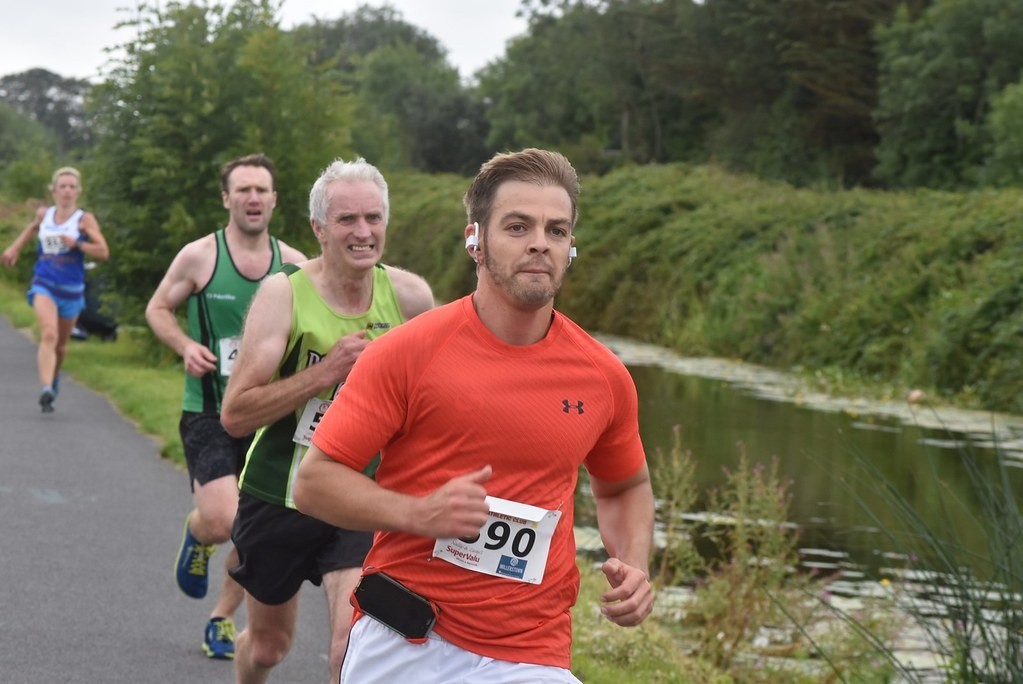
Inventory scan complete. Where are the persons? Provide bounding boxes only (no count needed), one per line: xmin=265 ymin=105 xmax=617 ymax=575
xmin=1 ymin=167 xmax=110 ymax=413
xmin=143 ymin=152 xmax=309 ymax=660
xmin=290 ymin=146 xmax=654 ymax=684
xmin=220 ymin=159 xmax=432 ymax=684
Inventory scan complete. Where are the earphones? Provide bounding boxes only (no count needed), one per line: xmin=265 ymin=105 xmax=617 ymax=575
xmin=468 ymin=223 xmax=479 ymax=253
xmin=569 ymin=244 xmax=577 ymax=264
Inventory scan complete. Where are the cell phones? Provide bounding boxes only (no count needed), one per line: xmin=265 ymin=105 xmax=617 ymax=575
xmin=349 ymin=567 xmax=440 ymax=645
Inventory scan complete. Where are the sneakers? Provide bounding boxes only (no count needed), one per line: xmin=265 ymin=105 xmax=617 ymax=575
xmin=173 ymin=510 xmax=217 ymax=601
xmin=37 ymin=387 xmax=55 ymax=412
xmin=51 ymin=376 xmax=62 ymax=398
xmin=202 ymin=619 xmax=237 ymax=660
